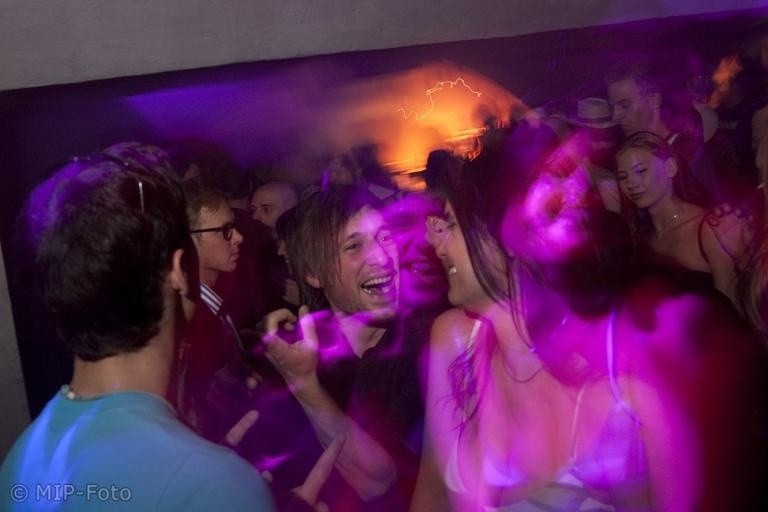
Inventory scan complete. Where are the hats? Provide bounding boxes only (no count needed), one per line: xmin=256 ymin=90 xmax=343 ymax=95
xmin=567 ymin=97 xmax=618 ymax=130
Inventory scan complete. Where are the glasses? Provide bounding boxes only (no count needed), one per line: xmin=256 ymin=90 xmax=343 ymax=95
xmin=73 ymin=150 xmax=156 ymax=212
xmin=191 ymin=223 xmax=233 ymax=241
